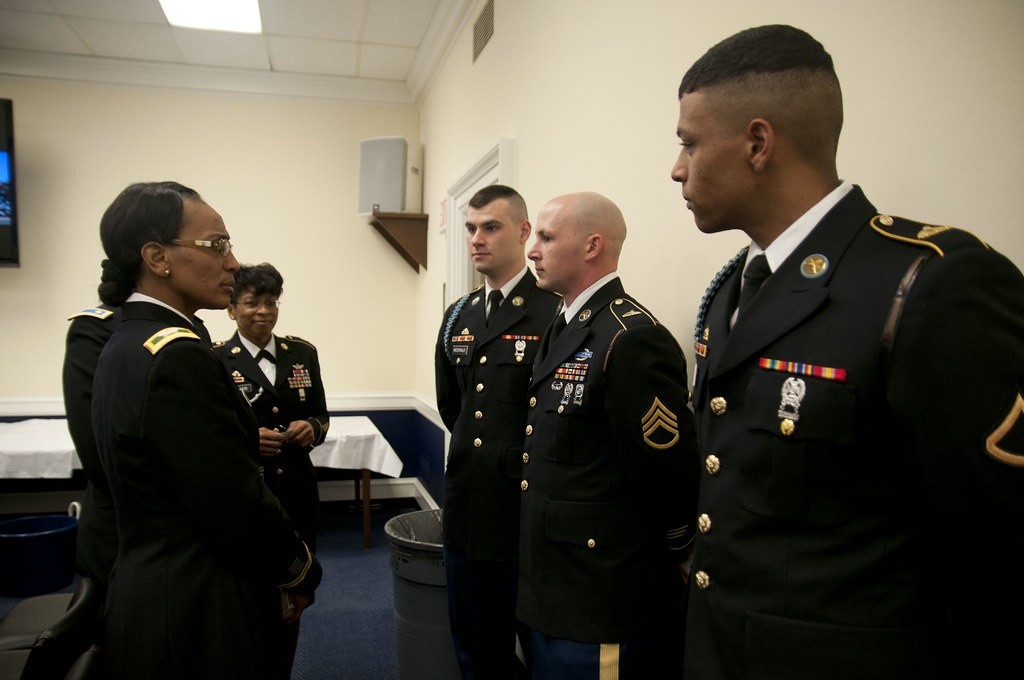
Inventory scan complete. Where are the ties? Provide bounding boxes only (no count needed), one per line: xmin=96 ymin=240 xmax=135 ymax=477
xmin=547 ymin=311 xmax=567 ymax=356
xmin=486 ymin=289 xmax=504 ymax=328
xmin=739 ymin=254 xmax=770 ymax=320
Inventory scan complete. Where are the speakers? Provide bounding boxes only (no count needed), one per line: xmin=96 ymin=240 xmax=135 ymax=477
xmin=357 ymin=136 xmax=424 ymax=216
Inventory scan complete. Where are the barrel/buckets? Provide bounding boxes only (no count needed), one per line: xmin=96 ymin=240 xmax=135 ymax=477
xmin=0 ymin=502 xmax=82 ymax=595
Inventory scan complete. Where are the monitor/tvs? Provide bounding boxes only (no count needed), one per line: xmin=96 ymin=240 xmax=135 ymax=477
xmin=0 ymin=98 xmax=21 ymax=267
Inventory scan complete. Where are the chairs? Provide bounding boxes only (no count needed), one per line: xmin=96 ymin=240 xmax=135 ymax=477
xmin=1 ymin=573 xmax=105 ymax=680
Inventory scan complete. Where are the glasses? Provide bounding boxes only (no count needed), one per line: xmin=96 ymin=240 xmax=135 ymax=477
xmin=235 ymin=299 xmax=282 ymax=311
xmin=163 ymin=236 xmax=231 ymax=256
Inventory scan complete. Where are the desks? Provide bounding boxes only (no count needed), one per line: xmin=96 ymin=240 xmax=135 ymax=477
xmin=1 ymin=411 xmax=402 ymax=551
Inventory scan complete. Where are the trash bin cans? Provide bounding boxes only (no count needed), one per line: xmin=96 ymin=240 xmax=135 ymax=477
xmin=0 ymin=515 xmax=82 ymax=624
xmin=384 ymin=507 xmax=526 ymax=680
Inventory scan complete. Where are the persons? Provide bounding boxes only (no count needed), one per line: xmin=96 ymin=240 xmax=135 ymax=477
xmin=92 ymin=181 xmax=324 ymax=679
xmin=435 ymin=184 xmax=563 ymax=680
xmin=672 ymin=25 xmax=1024 ymax=679
xmin=515 ymin=190 xmax=690 ymax=680
xmin=212 ymin=263 xmax=330 ymax=556
xmin=62 ymin=306 xmax=121 ymax=596
